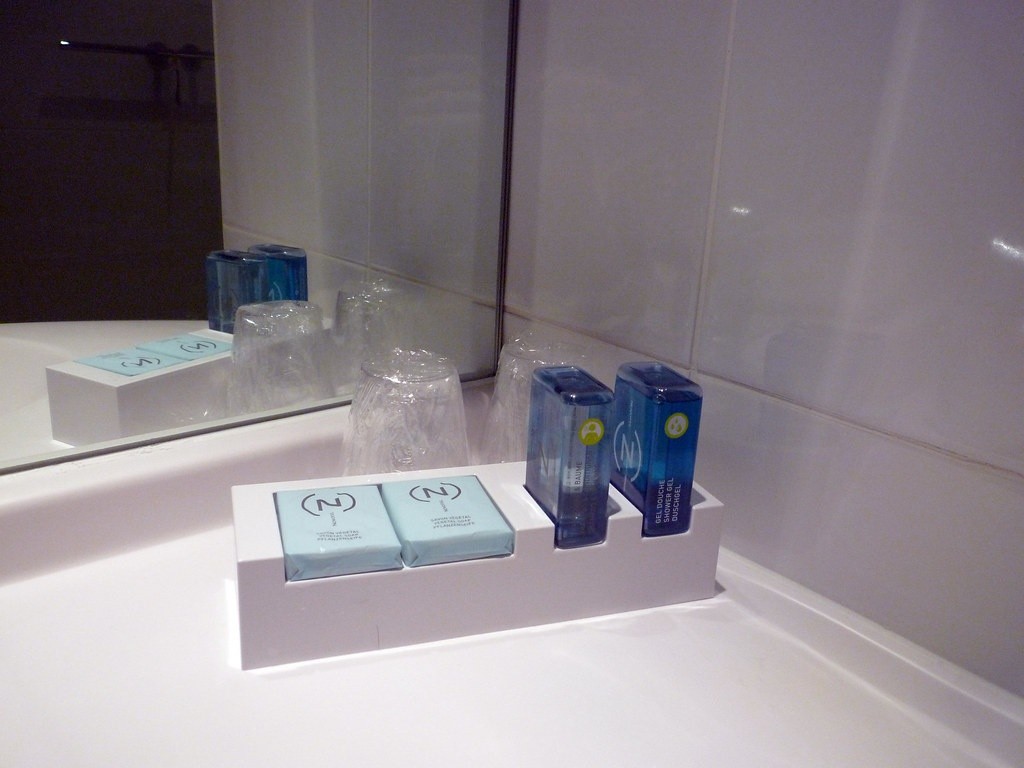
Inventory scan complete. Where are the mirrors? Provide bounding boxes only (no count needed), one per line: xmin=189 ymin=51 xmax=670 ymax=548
xmin=0 ymin=0 xmax=523 ymax=476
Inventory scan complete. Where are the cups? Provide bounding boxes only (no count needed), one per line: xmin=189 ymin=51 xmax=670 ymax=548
xmin=230 ymin=301 xmax=339 ymax=415
xmin=335 ymin=280 xmax=424 ymax=391
xmin=479 ymin=339 xmax=594 ymax=464
xmin=342 ymin=351 xmax=473 ymax=475
